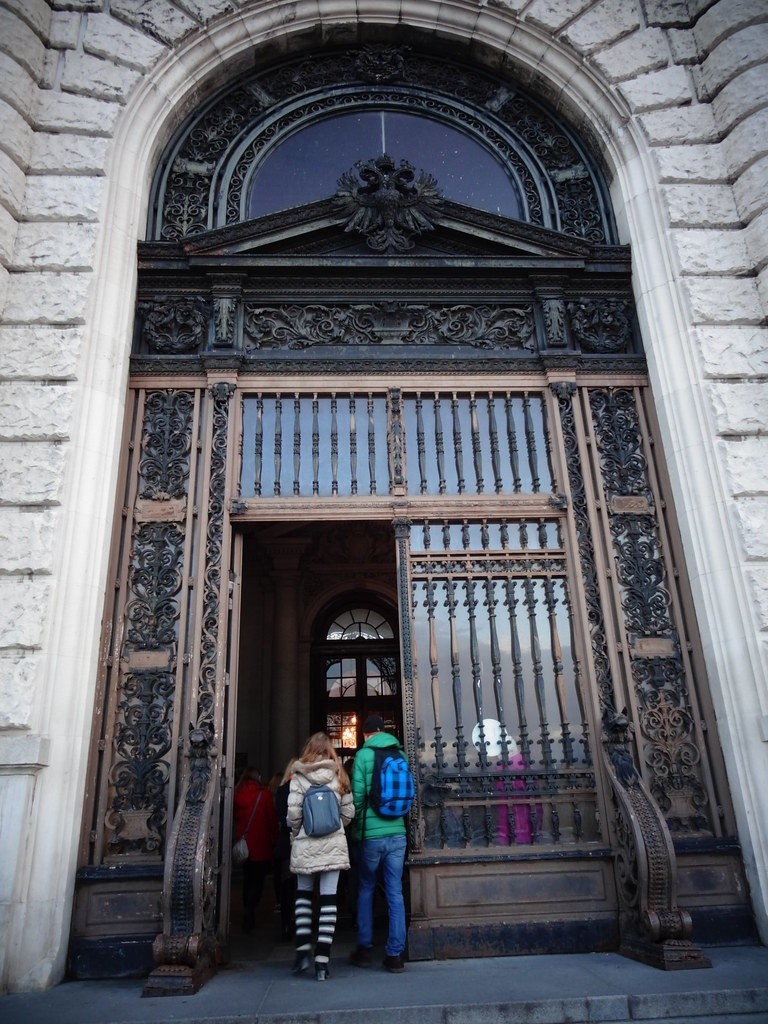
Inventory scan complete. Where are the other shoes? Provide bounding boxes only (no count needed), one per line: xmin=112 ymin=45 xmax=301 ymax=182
xmin=383 ymin=956 xmax=405 ymax=973
xmin=351 ymin=950 xmax=371 ymax=967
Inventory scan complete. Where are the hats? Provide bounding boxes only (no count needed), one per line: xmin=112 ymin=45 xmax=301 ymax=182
xmin=362 ymin=716 xmax=384 ymax=733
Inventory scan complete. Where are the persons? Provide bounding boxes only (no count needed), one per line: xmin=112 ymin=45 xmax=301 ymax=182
xmin=234 ymin=761 xmax=278 ymax=932
xmin=350 ymin=716 xmax=408 ymax=973
xmin=280 ymin=733 xmax=356 ymax=982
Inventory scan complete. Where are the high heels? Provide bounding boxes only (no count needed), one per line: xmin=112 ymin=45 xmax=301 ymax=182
xmin=298 ymin=951 xmax=311 ymax=970
xmin=314 ymin=962 xmax=330 ymax=981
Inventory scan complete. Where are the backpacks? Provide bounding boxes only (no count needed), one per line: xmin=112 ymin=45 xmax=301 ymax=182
xmin=302 ymin=784 xmax=341 ymax=838
xmin=366 ymin=744 xmax=415 ymax=820
